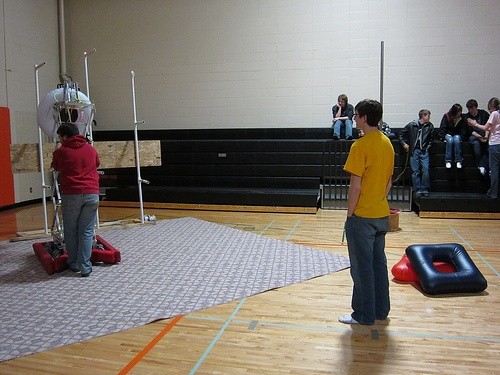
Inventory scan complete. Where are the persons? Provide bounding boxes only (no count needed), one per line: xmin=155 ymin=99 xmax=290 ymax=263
xmin=332 ymin=94 xmax=355 ymax=140
xmin=399 ymin=109 xmax=435 ymax=197
xmin=438 ymin=103 xmax=466 ymax=169
xmin=463 ymin=98 xmax=491 ymax=175
xmin=337 ymin=99 xmax=396 ymax=325
xmin=49 ymin=123 xmax=100 ymax=278
xmin=467 ymin=96 xmax=500 ymax=199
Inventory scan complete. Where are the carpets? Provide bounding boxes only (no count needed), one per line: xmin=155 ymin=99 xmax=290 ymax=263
xmin=0 ymin=217 xmax=350 ymax=363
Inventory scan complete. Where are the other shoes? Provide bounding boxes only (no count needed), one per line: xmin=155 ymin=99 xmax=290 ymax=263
xmin=333 ymin=135 xmax=340 ymax=140
xmin=81 ymin=267 xmax=92 ymax=277
xmin=346 ymin=135 xmax=353 ymax=140
xmin=478 ymin=167 xmax=487 ymax=176
xmin=338 ymin=314 xmax=359 ymax=324
xmin=446 ymin=161 xmax=451 ymax=168
xmin=457 ymin=162 xmax=462 ymax=168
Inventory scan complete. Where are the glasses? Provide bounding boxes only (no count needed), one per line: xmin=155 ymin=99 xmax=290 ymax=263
xmin=352 ymin=113 xmax=359 ymax=118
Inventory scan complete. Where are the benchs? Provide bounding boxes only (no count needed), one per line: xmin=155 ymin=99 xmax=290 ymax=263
xmin=90 ymin=127 xmax=500 ymax=212
